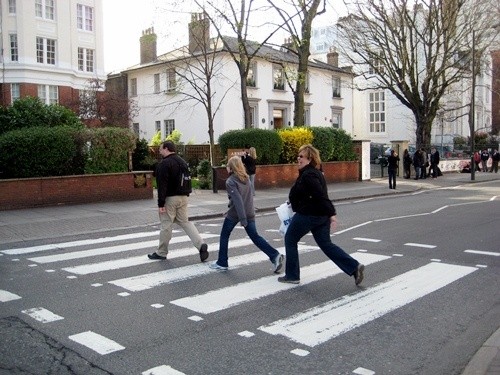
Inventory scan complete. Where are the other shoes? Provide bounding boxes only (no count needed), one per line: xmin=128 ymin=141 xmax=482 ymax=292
xmin=273 ymin=253 xmax=284 ymax=272
xmin=148 ymin=251 xmax=167 ymax=259
xmin=200 ymin=242 xmax=209 ymax=261
xmin=278 ymin=274 xmax=300 ymax=284
xmin=208 ymin=260 xmax=228 ymax=271
xmin=354 ymin=263 xmax=364 ymax=285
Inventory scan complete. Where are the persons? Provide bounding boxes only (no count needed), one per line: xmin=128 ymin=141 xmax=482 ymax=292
xmin=241 ymin=146 xmax=257 ymax=196
xmin=277 ymin=144 xmax=366 ymax=285
xmin=207 ymin=156 xmax=285 ymax=274
xmin=148 ymin=140 xmax=209 ymax=263
xmin=463 ymin=148 xmax=500 ymax=173
xmin=402 ymin=148 xmax=413 ymax=179
xmin=387 ymin=149 xmax=400 ymax=190
xmin=412 ymin=147 xmax=443 ymax=181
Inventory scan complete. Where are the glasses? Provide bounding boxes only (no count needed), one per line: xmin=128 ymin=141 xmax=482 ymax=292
xmin=296 ymin=154 xmax=306 ymax=158
